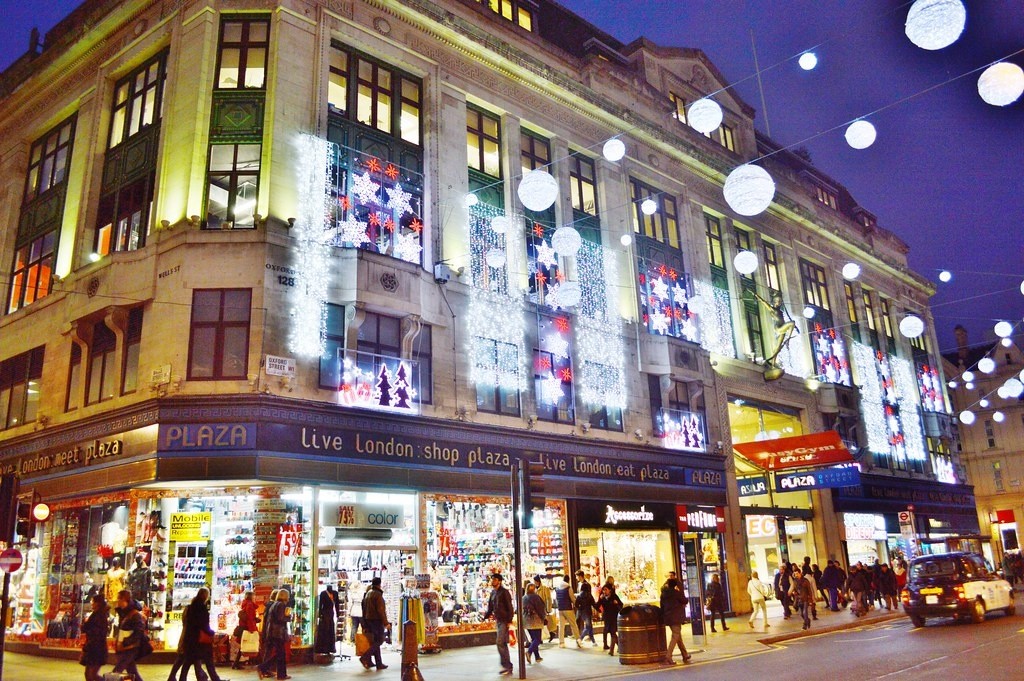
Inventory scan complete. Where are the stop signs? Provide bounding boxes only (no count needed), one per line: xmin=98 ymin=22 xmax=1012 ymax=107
xmin=0 ymin=548 xmax=22 ymax=574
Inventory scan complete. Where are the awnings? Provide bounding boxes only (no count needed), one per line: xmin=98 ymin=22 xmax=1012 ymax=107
xmin=732 ymin=430 xmax=853 ymax=472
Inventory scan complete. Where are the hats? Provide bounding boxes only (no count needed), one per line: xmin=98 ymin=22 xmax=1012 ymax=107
xmin=534 ymin=575 xmax=541 ymax=584
xmin=581 ymin=583 xmax=590 ymax=592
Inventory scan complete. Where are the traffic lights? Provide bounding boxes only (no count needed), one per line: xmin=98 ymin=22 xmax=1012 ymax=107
xmin=16 ymin=503 xmax=36 ymax=548
xmin=33 ymin=503 xmax=50 ymax=521
xmin=519 ymin=456 xmax=548 ymax=531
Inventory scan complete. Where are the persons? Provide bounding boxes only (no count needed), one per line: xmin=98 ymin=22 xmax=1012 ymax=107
xmin=106 ymin=553 xmax=150 ymax=605
xmin=79 ymin=595 xmax=108 ymax=681
xmin=774 ymin=556 xmax=907 ymax=631
xmin=746 ymin=571 xmax=770 ymax=628
xmin=232 ymin=589 xmax=293 ymax=680
xmin=1001 ymin=550 xmax=1024 ymax=587
xmin=166 ymin=588 xmax=231 ymax=681
xmin=315 ymin=585 xmax=340 ymax=653
xmin=522 ymin=570 xmax=624 ymax=663
xmin=706 ymin=574 xmax=729 ymax=632
xmin=111 ymin=591 xmax=153 ymax=681
xmin=594 ymin=556 xmax=607 ymax=583
xmin=482 ymin=573 xmax=515 ymax=674
xmin=660 ymin=571 xmax=692 ymax=664
xmin=348 ymin=577 xmax=391 ymax=670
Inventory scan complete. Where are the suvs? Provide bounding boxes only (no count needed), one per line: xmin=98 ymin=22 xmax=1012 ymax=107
xmin=900 ymin=550 xmax=1015 ymax=627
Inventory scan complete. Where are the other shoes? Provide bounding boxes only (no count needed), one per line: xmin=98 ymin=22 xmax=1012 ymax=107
xmin=499 ymin=667 xmax=513 ymax=674
xmin=360 ymin=655 xmax=371 ymax=669
xmin=536 ymin=657 xmax=543 ymax=663
xmin=377 ymin=664 xmax=389 ymax=670
xmin=527 ymin=594 xmax=896 ymax=664
xmin=256 ymin=666 xmax=263 ymax=679
xmin=277 ymin=674 xmax=291 ymax=680
xmin=264 ymin=670 xmax=275 ymax=677
xmin=526 ymin=652 xmax=532 ymax=664
xmin=232 ymin=663 xmax=245 ymax=670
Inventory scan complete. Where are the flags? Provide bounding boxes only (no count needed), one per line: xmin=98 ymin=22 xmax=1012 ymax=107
xmin=898 ymin=511 xmax=913 ymax=538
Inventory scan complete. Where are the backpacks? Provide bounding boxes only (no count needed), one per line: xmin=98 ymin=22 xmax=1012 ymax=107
xmin=850 ymin=592 xmax=867 ymax=618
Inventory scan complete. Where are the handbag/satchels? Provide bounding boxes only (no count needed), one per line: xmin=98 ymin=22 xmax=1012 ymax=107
xmin=525 ymin=625 xmax=551 ymax=642
xmin=240 ymin=630 xmax=259 ymax=653
xmin=233 ymin=626 xmax=248 ymax=637
xmin=704 ymin=597 xmax=712 ymax=609
xmin=546 ymin=611 xmax=557 ymax=632
xmin=355 ymin=628 xmax=374 ymax=656
xmin=764 ymin=595 xmax=767 ymax=601
xmin=197 ymin=631 xmax=215 ymax=646
xmin=792 ymin=594 xmax=799 ymax=609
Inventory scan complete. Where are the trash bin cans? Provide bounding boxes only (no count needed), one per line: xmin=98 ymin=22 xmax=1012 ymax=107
xmin=617 ymin=605 xmax=668 ymax=665
